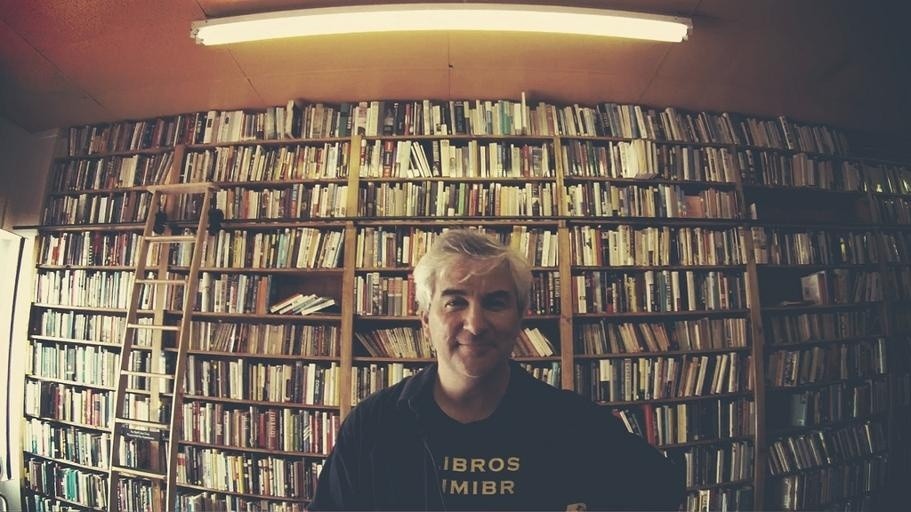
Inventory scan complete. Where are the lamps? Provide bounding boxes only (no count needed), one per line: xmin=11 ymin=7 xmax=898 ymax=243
xmin=189 ymin=0 xmax=694 ymax=47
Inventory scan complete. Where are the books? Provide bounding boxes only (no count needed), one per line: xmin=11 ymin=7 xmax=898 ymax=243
xmin=353 ymin=97 xmax=561 ymax=399
xmin=561 ymin=101 xmax=911 ymax=512
xmin=24 ymin=89 xmax=360 ymax=512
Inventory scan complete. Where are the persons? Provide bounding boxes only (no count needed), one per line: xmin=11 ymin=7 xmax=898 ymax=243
xmin=305 ymin=231 xmax=681 ymax=512
